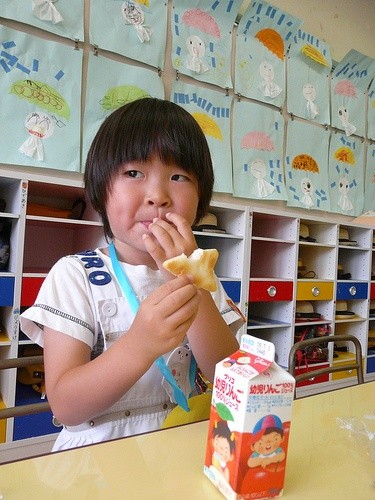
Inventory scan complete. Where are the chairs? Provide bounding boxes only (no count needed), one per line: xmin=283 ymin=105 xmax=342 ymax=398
xmin=288 ymin=335 xmax=364 ymax=399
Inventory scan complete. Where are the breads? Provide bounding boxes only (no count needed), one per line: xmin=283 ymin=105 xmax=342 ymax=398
xmin=161 ymin=248 xmax=220 ymax=292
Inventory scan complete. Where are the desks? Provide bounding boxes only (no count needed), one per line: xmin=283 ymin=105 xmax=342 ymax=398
xmin=0 ymin=380 xmax=375 ymax=500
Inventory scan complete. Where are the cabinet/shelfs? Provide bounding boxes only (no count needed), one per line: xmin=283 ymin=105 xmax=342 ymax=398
xmin=0 ymin=169 xmax=375 ymax=463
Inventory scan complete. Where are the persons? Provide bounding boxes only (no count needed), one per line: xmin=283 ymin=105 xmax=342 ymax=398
xmin=19 ymin=97 xmax=246 ymax=452
